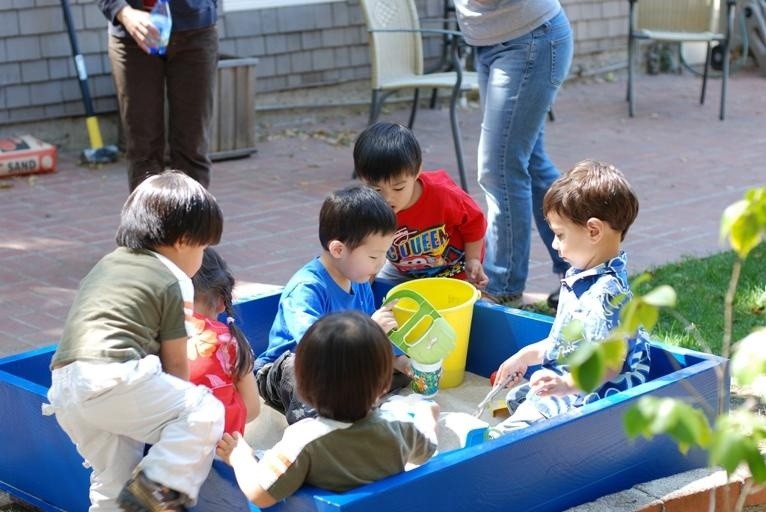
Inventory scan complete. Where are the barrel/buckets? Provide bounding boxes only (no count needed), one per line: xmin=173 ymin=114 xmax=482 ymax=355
xmin=384 ymin=276 xmax=480 ymax=388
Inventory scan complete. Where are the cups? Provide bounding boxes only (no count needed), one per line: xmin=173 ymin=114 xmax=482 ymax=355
xmin=411 ymin=358 xmax=442 ymax=399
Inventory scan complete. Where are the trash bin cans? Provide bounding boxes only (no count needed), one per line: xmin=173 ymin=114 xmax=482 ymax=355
xmin=162 ymin=53 xmax=260 ymax=166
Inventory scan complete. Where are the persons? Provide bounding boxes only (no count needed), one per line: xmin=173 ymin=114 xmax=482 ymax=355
xmin=215 ymin=310 xmax=440 ymax=509
xmin=96 ymin=0 xmax=221 ymax=199
xmin=252 ymin=185 xmax=415 ymax=426
xmin=351 ymin=120 xmax=490 ymax=289
xmin=487 ymin=159 xmax=651 ymax=440
xmin=449 ymin=0 xmax=575 ymax=306
xmin=43 ymin=170 xmax=226 ymax=512
xmin=180 ymin=246 xmax=260 ymax=453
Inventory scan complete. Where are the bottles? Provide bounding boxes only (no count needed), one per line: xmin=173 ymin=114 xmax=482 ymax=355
xmin=143 ymin=1 xmax=173 ymax=56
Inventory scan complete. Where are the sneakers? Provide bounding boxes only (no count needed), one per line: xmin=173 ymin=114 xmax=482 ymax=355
xmin=547 ymin=272 xmax=567 ymax=309
xmin=115 ymin=470 xmax=188 ymax=512
xmin=480 ymin=292 xmax=523 ymax=309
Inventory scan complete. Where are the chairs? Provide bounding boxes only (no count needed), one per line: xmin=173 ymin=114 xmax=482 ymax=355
xmin=621 ymin=0 xmax=737 ymax=121
xmin=429 ymin=0 xmax=561 ymax=123
xmin=356 ymin=0 xmax=484 ymax=200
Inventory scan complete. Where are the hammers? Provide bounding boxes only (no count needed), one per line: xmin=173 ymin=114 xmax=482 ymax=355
xmin=60 ymin=0 xmax=119 ymax=165
